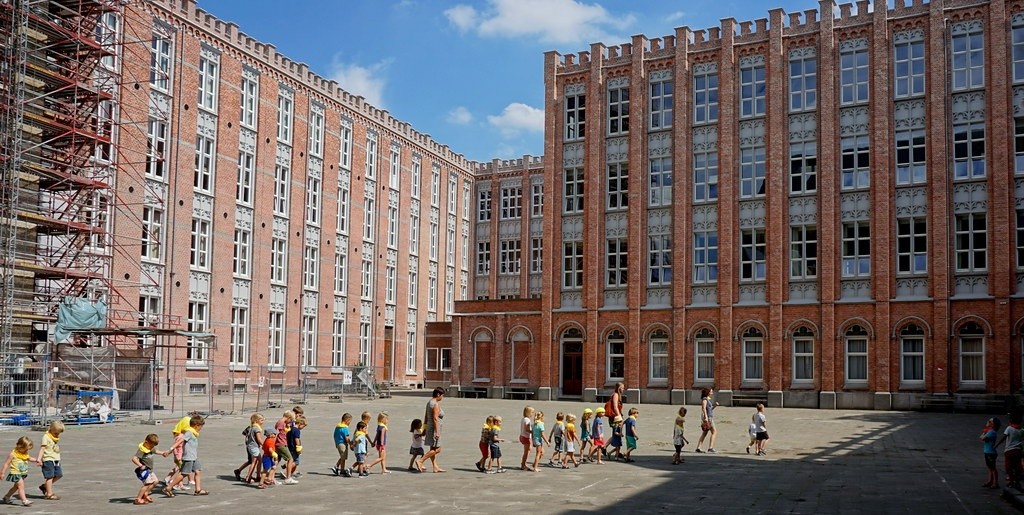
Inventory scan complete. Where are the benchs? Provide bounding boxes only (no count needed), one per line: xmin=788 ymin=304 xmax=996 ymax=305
xmin=506 ymin=392 xmax=535 ymax=400
xmin=596 ymin=394 xmax=628 ymax=403
xmin=458 ymin=390 xmax=486 ymax=398
xmin=966 ymin=404 xmax=997 ymax=414
xmin=921 ymin=399 xmax=956 ymax=414
xmin=730 ymin=398 xmax=767 ymax=407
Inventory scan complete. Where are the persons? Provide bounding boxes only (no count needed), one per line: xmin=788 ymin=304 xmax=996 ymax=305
xmin=519 ymin=382 xmax=640 ymax=472
xmin=331 ymin=411 xmax=391 ymax=478
xmin=695 ymin=387 xmax=719 ymax=453
xmin=747 ymin=404 xmax=768 ymax=456
xmin=36 ymin=420 xmax=65 ymax=500
xmin=475 ymin=415 xmax=508 ymax=474
xmin=132 ymin=414 xmax=210 ymax=505
xmin=671 ymin=407 xmax=689 ymax=465
xmin=0 ymin=436 xmax=39 ymax=506
xmin=409 ymin=387 xmax=447 ymax=473
xmin=234 ymin=406 xmax=308 ymax=489
xmin=979 ymin=414 xmax=1024 ymax=490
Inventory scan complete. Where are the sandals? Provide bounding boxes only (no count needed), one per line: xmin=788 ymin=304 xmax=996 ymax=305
xmin=265 ymin=480 xmax=275 ymax=485
xmin=23 ymin=500 xmax=33 ymax=505
xmin=981 ymin=482 xmax=995 ymax=487
xmin=134 ymin=499 xmax=148 ymax=504
xmin=39 ymin=484 xmax=47 ymax=495
xmin=258 ymin=484 xmax=267 ymax=488
xmin=3 ymin=496 xmax=14 ymax=504
xmin=989 ymin=484 xmax=1000 ymax=489
xmin=45 ymin=493 xmax=60 ymax=500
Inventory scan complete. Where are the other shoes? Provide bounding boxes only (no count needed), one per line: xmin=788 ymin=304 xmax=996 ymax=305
xmin=1007 ymin=481 xmax=1014 ymax=488
xmin=159 ymin=478 xmax=212 ymax=499
xmin=474 ymin=453 xmax=635 ymax=475
xmin=1015 ymin=482 xmax=1021 ymax=489
xmin=695 ymin=448 xmax=705 ymax=453
xmin=330 ymin=466 xmax=392 ymax=478
xmin=707 ymin=448 xmax=717 ymax=453
xmin=234 ymin=469 xmax=244 ymax=480
xmin=283 ymin=478 xmax=299 ymax=484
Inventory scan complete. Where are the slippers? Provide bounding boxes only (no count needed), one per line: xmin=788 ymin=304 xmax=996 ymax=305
xmin=143 ymin=495 xmax=153 ymax=502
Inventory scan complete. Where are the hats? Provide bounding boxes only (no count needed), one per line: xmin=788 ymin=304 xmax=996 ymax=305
xmin=596 ymin=407 xmax=606 ymax=413
xmin=613 ymin=416 xmax=622 ymax=422
xmin=585 ymin=408 xmax=592 ymax=413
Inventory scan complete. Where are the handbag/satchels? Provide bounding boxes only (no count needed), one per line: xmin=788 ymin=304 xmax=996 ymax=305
xmin=701 ymin=421 xmax=710 ymax=431
xmin=605 ymin=392 xmax=622 ymax=417
